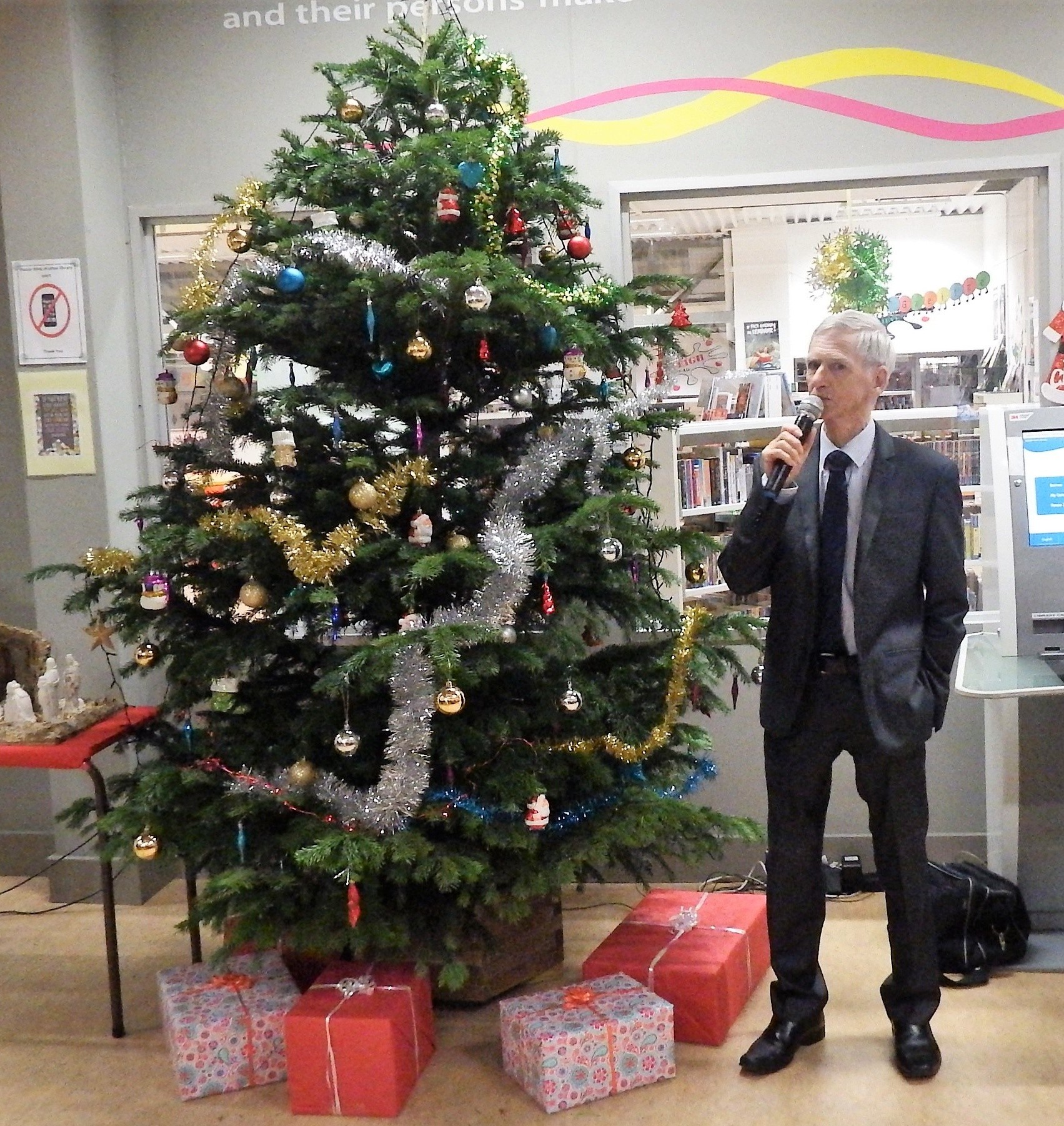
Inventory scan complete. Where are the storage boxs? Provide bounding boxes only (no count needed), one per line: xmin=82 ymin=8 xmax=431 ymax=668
xmin=284 ymin=960 xmax=436 ymax=1119
xmin=583 ymin=888 xmax=770 ymax=1047
xmin=156 ymin=949 xmax=301 ymax=1101
xmin=499 ymin=972 xmax=676 ymax=1114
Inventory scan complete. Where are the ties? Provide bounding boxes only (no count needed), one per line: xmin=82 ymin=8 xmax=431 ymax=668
xmin=818 ymin=450 xmax=849 ymax=696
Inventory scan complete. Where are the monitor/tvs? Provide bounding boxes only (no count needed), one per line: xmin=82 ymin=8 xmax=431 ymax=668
xmin=1005 ymin=409 xmax=1063 ymax=660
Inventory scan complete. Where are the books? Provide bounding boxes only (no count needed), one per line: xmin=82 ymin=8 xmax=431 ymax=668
xmin=677 ymin=449 xmax=754 ymax=511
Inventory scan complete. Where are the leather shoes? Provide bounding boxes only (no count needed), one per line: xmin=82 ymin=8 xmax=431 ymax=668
xmin=739 ymin=1014 xmax=826 ymax=1076
xmin=891 ymin=1017 xmax=941 ymax=1083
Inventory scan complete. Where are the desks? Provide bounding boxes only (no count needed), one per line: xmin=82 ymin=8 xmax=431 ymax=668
xmin=0 ymin=705 xmax=202 ymax=1039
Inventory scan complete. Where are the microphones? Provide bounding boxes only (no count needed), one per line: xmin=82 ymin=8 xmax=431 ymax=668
xmin=763 ymin=395 xmax=824 ymax=500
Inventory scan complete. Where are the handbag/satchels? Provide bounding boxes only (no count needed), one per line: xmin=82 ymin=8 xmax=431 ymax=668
xmin=926 ymin=857 xmax=1028 ymax=989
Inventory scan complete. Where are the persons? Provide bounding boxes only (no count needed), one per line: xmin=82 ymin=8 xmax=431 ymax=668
xmin=717 ymin=310 xmax=969 ymax=1079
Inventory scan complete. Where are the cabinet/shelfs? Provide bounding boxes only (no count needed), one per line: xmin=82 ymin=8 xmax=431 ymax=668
xmin=630 ymin=406 xmax=983 ymax=632
